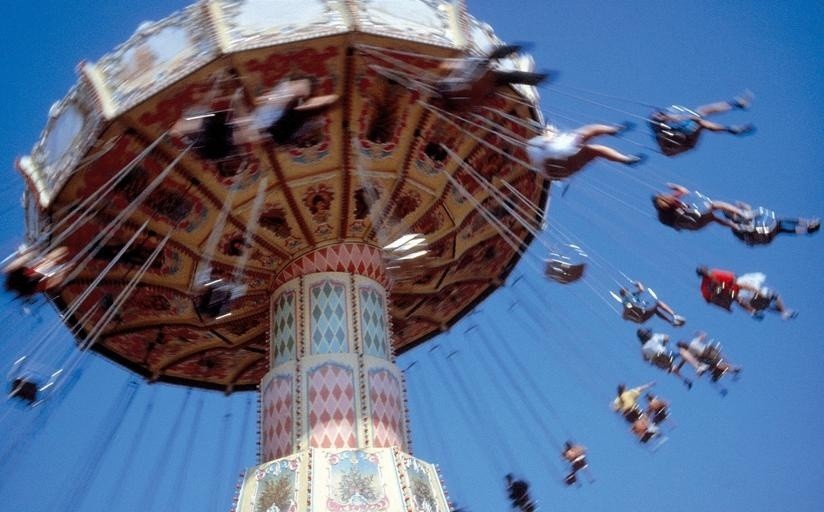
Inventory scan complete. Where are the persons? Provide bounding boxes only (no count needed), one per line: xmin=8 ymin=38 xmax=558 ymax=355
xmin=619 ymin=279 xmax=744 ymax=376
xmin=505 ymin=474 xmax=533 ymax=511
xmin=258 ymin=78 xmax=338 ymax=120
xmin=696 ymin=263 xmax=800 ymax=320
xmin=4 ymin=245 xmax=69 ymax=295
xmin=610 ymin=381 xmax=664 ymax=439
xmin=653 ymin=184 xmax=820 ymax=242
xmin=528 ymin=119 xmax=647 ymax=179
xmin=443 ymin=45 xmax=550 ymax=89
xmin=562 ymin=440 xmax=585 ymax=459
xmin=654 ymin=92 xmax=758 ymax=138
